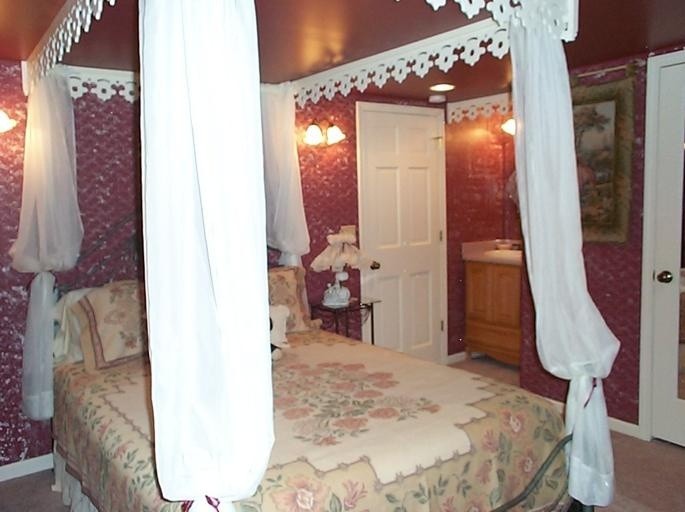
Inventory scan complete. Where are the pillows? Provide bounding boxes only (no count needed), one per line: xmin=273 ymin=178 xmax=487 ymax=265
xmin=270 ymin=304 xmax=290 ymax=347
xmin=269 ymin=263 xmax=312 ymax=333
xmin=66 ymin=280 xmax=147 ymax=373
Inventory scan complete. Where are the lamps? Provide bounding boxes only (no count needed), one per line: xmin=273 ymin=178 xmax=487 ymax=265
xmin=465 ymin=258 xmax=520 ymax=366
xmin=0 ymin=110 xmax=20 ymax=133
xmin=502 ymin=118 xmax=516 ymax=137
xmin=304 ymin=118 xmax=348 ymax=146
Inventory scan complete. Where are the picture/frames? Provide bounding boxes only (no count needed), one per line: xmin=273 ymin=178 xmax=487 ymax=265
xmin=571 ymin=77 xmax=636 ymax=242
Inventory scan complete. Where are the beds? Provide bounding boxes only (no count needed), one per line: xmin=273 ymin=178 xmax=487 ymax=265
xmin=48 ymin=265 xmax=570 ymax=512
